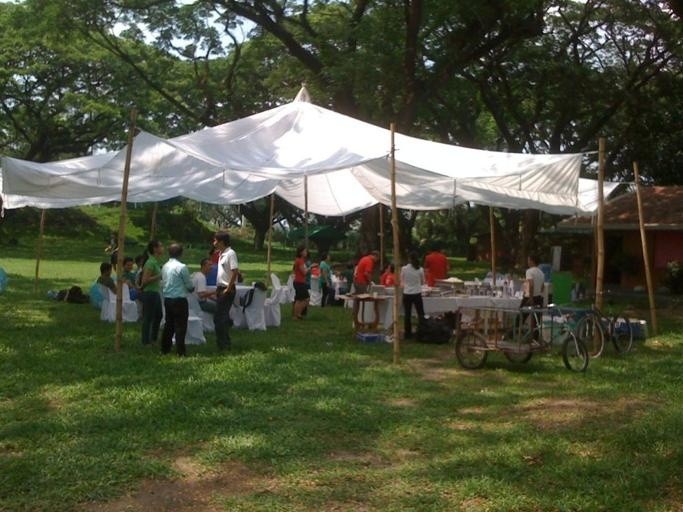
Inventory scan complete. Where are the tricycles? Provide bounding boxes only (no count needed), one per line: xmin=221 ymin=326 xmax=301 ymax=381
xmin=455 ymin=301 xmax=588 ymax=372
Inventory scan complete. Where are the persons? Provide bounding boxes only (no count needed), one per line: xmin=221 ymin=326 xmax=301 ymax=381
xmin=213 ymin=232 xmax=238 ymax=351
xmin=208 ymin=235 xmax=221 ymax=263
xmin=47 ymin=232 xmax=144 ymax=304
xmin=424 ymin=244 xmax=451 ymax=288
xmin=380 ymin=265 xmax=400 ymax=287
xmin=206 ymin=257 xmax=219 ymax=285
xmin=160 ymin=245 xmax=194 ymax=358
xmin=192 ymin=258 xmax=233 ymax=327
xmin=537 ymin=254 xmax=552 ymax=304
xmin=289 ymin=246 xmax=360 ymax=321
xmin=400 ymin=252 xmax=426 ymax=340
xmin=142 ymin=239 xmax=164 ymax=346
xmin=352 ymin=250 xmax=380 ymax=328
xmin=510 ymin=254 xmax=545 ymax=342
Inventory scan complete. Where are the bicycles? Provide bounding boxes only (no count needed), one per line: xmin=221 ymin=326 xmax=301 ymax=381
xmin=573 ymin=290 xmax=632 ymax=359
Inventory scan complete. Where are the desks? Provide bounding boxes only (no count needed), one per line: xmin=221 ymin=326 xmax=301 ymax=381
xmin=373 ymin=292 xmax=591 ymax=351
xmin=335 ymin=292 xmax=390 ymax=333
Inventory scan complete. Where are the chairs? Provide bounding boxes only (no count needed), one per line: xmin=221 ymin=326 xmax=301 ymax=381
xmin=96 ymin=273 xmax=347 ymax=346
